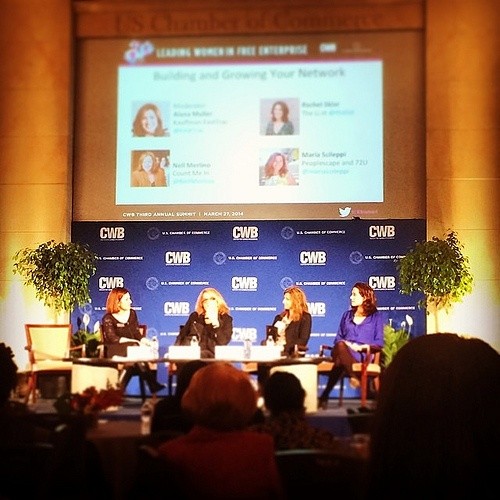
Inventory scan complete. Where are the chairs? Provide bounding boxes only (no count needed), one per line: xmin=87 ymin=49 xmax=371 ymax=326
xmin=24 ymin=324 xmax=72 ymax=404
xmin=319 ymin=345 xmax=380 ymax=406
xmin=275 ymin=446 xmax=334 ymax=491
xmin=98 ymin=325 xmax=148 ymax=404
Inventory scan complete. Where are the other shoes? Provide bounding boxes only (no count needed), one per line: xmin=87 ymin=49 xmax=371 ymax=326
xmin=149 ymin=382 xmax=165 ymax=393
xmin=350 ymin=377 xmax=360 ymax=389
xmin=318 ymin=397 xmax=328 ymax=409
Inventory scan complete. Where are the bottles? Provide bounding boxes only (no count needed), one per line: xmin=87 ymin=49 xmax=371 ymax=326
xmin=267 ymin=335 xmax=274 ymax=347
xmin=190 ymin=335 xmax=198 ymax=359
xmin=151 ymin=335 xmax=159 ymax=360
xmin=244 ymin=338 xmax=253 ymax=360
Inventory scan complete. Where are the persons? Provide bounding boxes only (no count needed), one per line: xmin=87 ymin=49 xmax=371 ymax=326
xmin=157 ymin=363 xmax=282 ymax=500
xmin=132 ymin=152 xmax=169 ymax=186
xmin=0 ymin=341 xmax=113 ymax=500
xmin=256 ymin=284 xmax=311 ymax=396
xmin=318 ymin=282 xmax=384 ymax=410
xmin=266 ymin=102 xmax=294 ymax=135
xmin=323 ymin=332 xmax=500 ymax=500
xmin=150 ymin=360 xmax=209 ymax=437
xmin=169 ymin=287 xmax=233 ymax=394
xmin=132 ymin=103 xmax=169 ymax=137
xmin=259 ymin=372 xmax=335 ymax=451
xmin=265 ymin=152 xmax=296 ymax=185
xmin=102 ymin=288 xmax=165 ymax=393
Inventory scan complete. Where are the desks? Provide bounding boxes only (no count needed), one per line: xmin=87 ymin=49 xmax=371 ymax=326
xmin=78 ymin=357 xmax=164 ymax=403
xmin=165 ymin=356 xmax=328 ymax=414
